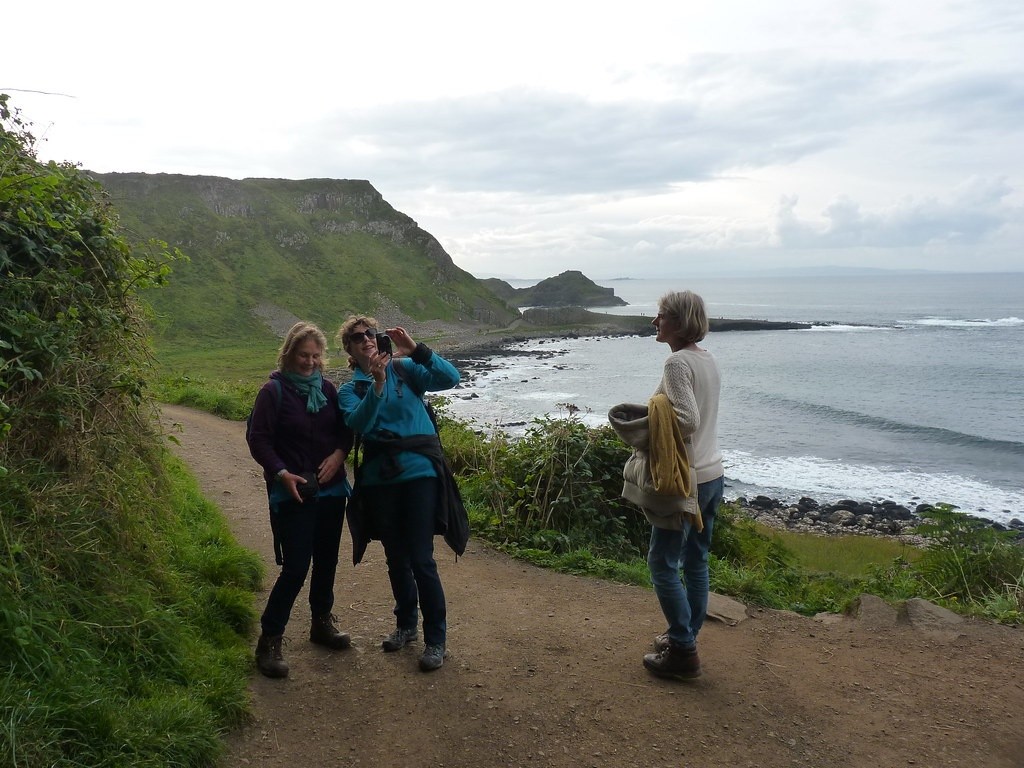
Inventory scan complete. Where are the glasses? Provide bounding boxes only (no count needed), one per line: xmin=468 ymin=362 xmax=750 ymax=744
xmin=350 ymin=328 xmax=377 ymax=344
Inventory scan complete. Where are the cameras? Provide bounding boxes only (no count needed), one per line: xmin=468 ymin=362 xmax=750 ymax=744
xmin=376 ymin=332 xmax=393 ymax=358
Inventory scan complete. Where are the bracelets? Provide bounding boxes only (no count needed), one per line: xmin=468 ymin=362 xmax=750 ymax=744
xmin=374 ymin=379 xmax=386 ymax=384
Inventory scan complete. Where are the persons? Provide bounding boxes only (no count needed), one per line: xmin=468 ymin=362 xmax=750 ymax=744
xmin=607 ymin=291 xmax=724 ymax=679
xmin=246 ymin=315 xmax=462 ymax=678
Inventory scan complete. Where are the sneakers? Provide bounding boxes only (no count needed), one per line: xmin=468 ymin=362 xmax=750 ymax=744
xmin=421 ymin=640 xmax=446 ymax=669
xmin=382 ymin=624 xmax=419 ymax=650
xmin=654 ymin=636 xmax=671 ymax=652
xmin=255 ymin=634 xmax=292 ymax=679
xmin=643 ymin=639 xmax=703 ymax=680
xmin=309 ymin=616 xmax=348 ymax=648
